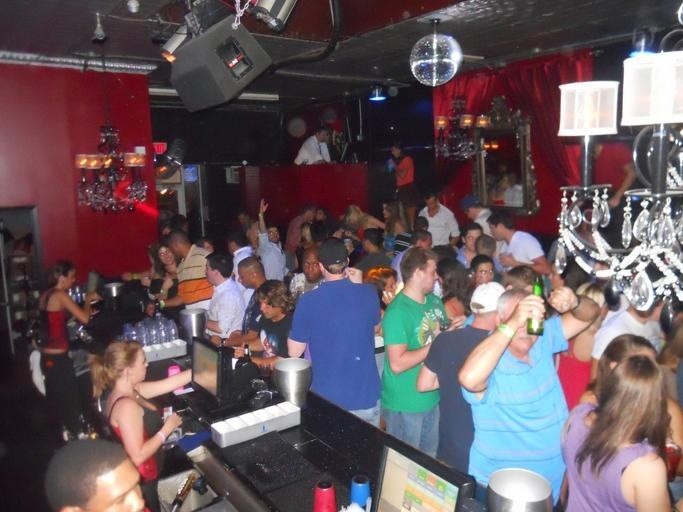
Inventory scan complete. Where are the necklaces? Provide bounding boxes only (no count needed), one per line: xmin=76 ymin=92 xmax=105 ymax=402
xmin=125 ymin=388 xmax=140 ymax=402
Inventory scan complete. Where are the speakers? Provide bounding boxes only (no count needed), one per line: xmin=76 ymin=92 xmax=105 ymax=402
xmin=170 ymin=15 xmax=272 ymax=112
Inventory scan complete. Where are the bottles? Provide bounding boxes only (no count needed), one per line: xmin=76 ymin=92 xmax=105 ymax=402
xmin=527 ymin=276 xmax=546 ymax=335
xmin=66 ymin=285 xmax=82 ymax=305
xmin=60 ymin=413 xmax=99 ymax=442
xmin=241 ymin=343 xmax=253 ymax=363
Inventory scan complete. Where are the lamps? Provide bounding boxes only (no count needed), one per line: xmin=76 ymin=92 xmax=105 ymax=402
xmin=369 ymin=81 xmax=388 ymax=103
xmin=250 ymin=1 xmax=299 ymax=34
xmin=158 ymin=23 xmax=194 ymax=66
xmin=68 ymin=116 xmax=149 ymax=213
xmin=540 ymin=0 xmax=683 ymax=334
xmin=428 ymin=80 xmax=492 ymax=163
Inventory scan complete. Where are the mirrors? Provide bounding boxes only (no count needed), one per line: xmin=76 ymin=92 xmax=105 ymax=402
xmin=468 ymin=92 xmax=540 ymax=219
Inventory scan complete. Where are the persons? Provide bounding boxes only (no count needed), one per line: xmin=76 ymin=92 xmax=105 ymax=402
xmin=3 ymin=228 xmax=14 ymax=273
xmin=86 ymin=344 xmax=192 ymax=512
xmin=43 ymin=439 xmax=151 ymax=512
xmin=38 ymin=260 xmax=105 ymax=441
xmin=151 ymin=177 xmax=683 ymax=512
xmin=389 ymin=145 xmax=420 ymax=224
xmin=294 ymin=126 xmax=333 ymax=165
xmin=14 ymin=233 xmax=31 ymax=256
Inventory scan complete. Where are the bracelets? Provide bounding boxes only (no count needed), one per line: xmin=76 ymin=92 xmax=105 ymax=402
xmin=157 ymin=431 xmax=167 ymax=443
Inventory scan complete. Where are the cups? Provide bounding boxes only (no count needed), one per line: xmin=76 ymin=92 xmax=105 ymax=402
xmin=124 ymin=314 xmax=177 ymax=346
xmin=485 ymin=467 xmax=552 ymax=512
xmin=272 ymin=357 xmax=311 ymax=410
xmin=349 ymin=474 xmax=371 ymax=507
xmin=663 ymin=443 xmax=681 ymax=483
xmin=313 ymin=480 xmax=337 ymax=512
xmin=168 ymin=366 xmax=183 ymax=392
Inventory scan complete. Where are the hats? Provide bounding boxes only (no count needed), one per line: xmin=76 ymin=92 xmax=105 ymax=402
xmin=469 ymin=281 xmax=505 ymax=313
xmin=364 ymin=228 xmax=384 ymax=244
xmin=460 ymin=196 xmax=481 ymax=211
xmin=317 ymin=236 xmax=349 ymax=263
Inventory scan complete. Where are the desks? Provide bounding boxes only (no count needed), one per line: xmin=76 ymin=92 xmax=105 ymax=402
xmin=256 ymin=160 xmax=374 ymax=225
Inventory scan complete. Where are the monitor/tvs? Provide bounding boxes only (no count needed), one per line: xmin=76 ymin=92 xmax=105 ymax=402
xmin=87 ymin=271 xmax=104 ymax=295
xmin=370 ymin=441 xmax=475 ymax=512
xmin=192 ymin=337 xmax=231 ymax=411
xmin=339 ymin=142 xmax=359 ymax=164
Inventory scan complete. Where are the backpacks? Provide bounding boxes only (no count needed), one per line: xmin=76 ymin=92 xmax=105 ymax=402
xmin=27 ymin=310 xmax=49 ymax=347
